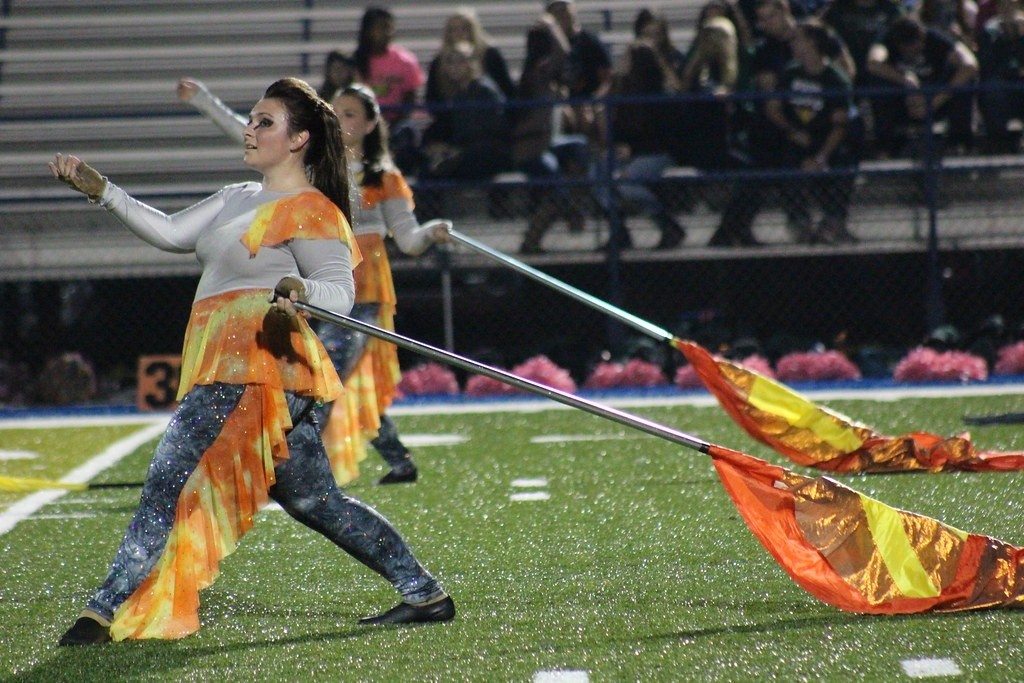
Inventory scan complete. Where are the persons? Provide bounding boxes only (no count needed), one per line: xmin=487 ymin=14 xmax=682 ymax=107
xmin=46 ymin=78 xmax=458 ymax=645
xmin=283 ymin=3 xmax=1024 ymax=262
xmin=174 ymin=80 xmax=424 ymax=489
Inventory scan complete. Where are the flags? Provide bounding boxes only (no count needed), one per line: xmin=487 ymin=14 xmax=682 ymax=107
xmin=704 ymin=443 xmax=1024 ymax=615
xmin=669 ymin=339 xmax=1022 ymax=480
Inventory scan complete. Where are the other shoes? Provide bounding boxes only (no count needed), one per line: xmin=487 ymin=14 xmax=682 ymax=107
xmin=655 ymin=223 xmax=685 ymax=249
xmin=596 ymin=234 xmax=633 ymax=250
xmin=357 ymin=592 xmax=456 ymax=627
xmin=519 ymin=241 xmax=547 ymax=255
xmin=706 ymin=226 xmax=859 ymax=245
xmin=57 ymin=609 xmax=112 ymax=648
xmin=376 ymin=466 xmax=417 ymax=484
